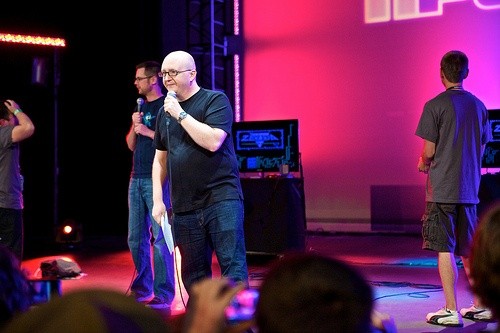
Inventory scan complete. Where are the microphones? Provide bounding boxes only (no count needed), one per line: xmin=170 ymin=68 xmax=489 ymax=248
xmin=166 ymin=91 xmax=177 ymax=126
xmin=137 ymin=98 xmax=144 ymax=113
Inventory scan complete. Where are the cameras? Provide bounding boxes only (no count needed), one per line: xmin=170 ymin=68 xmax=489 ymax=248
xmin=28 ymin=280 xmax=61 ymax=307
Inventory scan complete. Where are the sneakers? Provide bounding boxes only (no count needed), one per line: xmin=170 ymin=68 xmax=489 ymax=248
xmin=460 ymin=305 xmax=493 ymax=321
xmin=425 ymin=305 xmax=464 ymax=327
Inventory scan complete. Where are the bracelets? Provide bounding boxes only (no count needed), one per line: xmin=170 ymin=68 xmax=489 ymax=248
xmin=13 ymin=109 xmax=22 ymax=115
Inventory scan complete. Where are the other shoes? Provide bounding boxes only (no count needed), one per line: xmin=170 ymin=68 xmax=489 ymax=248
xmin=147 ymin=298 xmax=168 ymax=308
xmin=130 ymin=292 xmax=151 ymax=301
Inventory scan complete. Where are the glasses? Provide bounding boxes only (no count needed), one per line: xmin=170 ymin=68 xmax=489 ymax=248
xmin=136 ymin=76 xmax=151 ymax=82
xmin=158 ymin=69 xmax=191 ymax=78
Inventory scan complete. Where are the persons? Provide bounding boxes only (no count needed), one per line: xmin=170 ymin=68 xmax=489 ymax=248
xmin=415 ymin=49 xmax=492 ymax=326
xmin=0 ymin=99 xmax=35 ymax=262
xmin=0 ymin=251 xmax=398 ymax=333
xmin=126 ymin=63 xmax=176 ymax=308
xmin=468 ymin=202 xmax=500 ymax=333
xmin=152 ymin=50 xmax=249 ymax=312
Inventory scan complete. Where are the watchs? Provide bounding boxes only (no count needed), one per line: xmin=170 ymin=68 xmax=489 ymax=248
xmin=177 ymin=112 xmax=187 ymax=124
